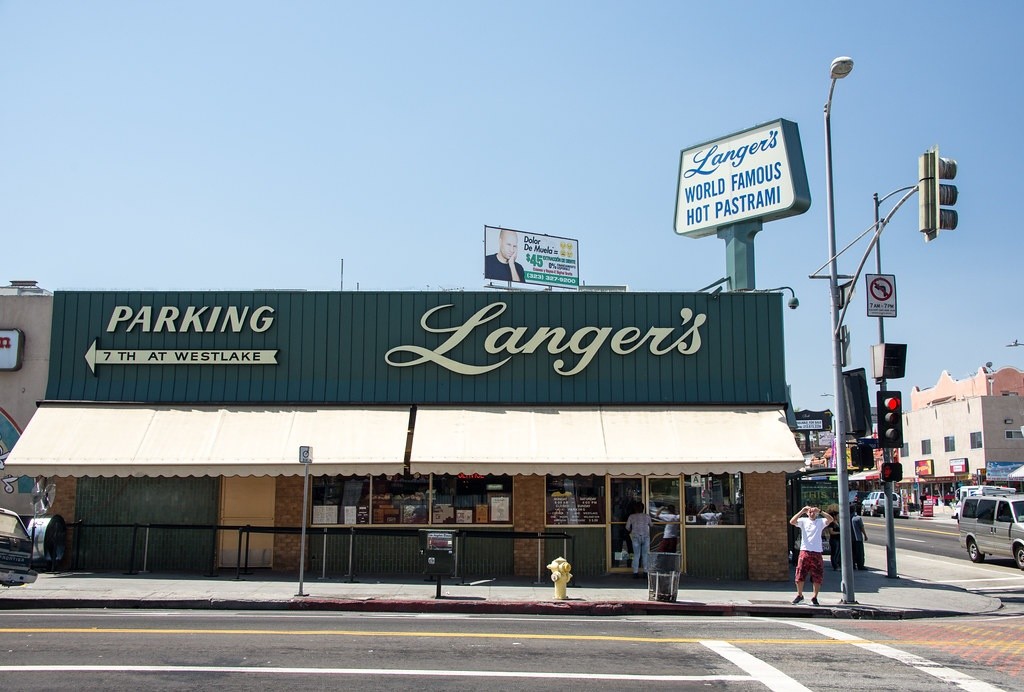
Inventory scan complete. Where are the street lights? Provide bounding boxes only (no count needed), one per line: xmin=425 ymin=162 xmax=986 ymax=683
xmin=824 ymin=56 xmax=855 ymax=606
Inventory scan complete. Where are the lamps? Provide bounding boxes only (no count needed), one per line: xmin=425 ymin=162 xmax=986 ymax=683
xmin=754 ymin=286 xmax=799 ymax=309
xmin=1004 ymin=418 xmax=1014 ymax=424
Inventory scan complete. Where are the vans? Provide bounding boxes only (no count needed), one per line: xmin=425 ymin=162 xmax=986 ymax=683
xmin=960 ymin=496 xmax=1024 ymax=569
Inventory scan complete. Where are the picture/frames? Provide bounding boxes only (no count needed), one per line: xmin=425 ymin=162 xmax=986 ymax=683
xmin=487 ymin=491 xmax=512 ymax=524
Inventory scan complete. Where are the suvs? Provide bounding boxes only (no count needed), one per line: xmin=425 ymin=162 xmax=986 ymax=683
xmin=861 ymin=490 xmax=900 ymax=517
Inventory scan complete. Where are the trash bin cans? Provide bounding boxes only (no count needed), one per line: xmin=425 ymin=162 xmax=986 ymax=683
xmin=648 ymin=551 xmax=684 ymax=604
xmin=417 ymin=528 xmax=466 ymax=579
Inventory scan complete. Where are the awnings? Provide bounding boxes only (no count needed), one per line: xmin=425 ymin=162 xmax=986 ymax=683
xmin=3 ymin=397 xmax=807 ymax=476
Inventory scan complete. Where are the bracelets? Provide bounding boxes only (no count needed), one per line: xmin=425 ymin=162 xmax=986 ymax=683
xmin=819 ymin=509 xmax=822 ymax=514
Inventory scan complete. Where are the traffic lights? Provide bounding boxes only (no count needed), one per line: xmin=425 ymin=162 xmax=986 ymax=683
xmin=876 ymin=391 xmax=903 ymax=448
xmin=917 ymin=145 xmax=959 ymax=244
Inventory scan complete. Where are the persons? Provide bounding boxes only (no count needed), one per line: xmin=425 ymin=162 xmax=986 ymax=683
xmin=551 ymin=483 xmax=572 ymax=516
xmin=919 ymin=489 xmax=927 ymax=515
xmin=827 ymin=510 xmax=841 ymax=571
xmin=655 ymin=505 xmax=681 ymax=554
xmin=789 ymin=504 xmax=834 ymax=606
xmin=485 ymin=229 xmax=526 ymax=282
xmin=848 ymin=506 xmax=868 ymax=570
xmin=697 ymin=503 xmax=725 ymax=525
xmin=626 ymin=502 xmax=653 ymax=578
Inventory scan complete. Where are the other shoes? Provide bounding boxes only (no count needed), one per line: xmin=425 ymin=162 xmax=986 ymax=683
xmin=811 ymin=598 xmax=819 ymax=606
xmin=858 ymin=566 xmax=868 ymax=571
xmin=793 ymin=595 xmax=804 ymax=604
xmin=834 ymin=566 xmax=841 ymax=571
xmin=633 ymin=568 xmax=638 ymax=573
xmin=853 ymin=564 xmax=856 ymax=570
xmin=643 ymin=568 xmax=648 ymax=573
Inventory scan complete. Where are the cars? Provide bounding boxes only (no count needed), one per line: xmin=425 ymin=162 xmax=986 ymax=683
xmin=0 ymin=508 xmax=38 ymax=587
